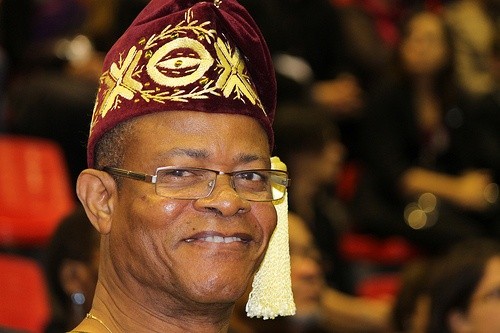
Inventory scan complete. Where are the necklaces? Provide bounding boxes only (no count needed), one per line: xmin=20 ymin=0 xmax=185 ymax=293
xmin=87 ymin=312 xmax=113 ymax=332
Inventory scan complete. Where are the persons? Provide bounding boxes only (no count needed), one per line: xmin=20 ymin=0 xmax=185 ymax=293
xmin=1 ymin=0 xmax=145 ymax=333
xmin=67 ymin=0 xmax=291 ymax=333
xmin=235 ymin=1 xmax=499 ymax=333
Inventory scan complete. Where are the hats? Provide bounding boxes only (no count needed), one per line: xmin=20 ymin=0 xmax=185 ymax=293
xmin=87 ymin=0 xmax=278 ymax=168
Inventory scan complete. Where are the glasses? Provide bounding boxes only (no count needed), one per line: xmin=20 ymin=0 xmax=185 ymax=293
xmin=101 ymin=166 xmax=291 ymax=202
